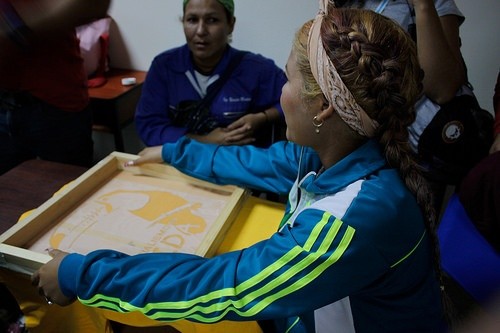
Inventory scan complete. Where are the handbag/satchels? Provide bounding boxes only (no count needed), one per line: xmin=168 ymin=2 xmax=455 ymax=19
xmin=74 ymin=16 xmax=116 ymax=84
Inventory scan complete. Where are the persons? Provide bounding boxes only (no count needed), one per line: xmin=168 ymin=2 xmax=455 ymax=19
xmin=135 ymin=0 xmax=288 ymax=147
xmin=0 ymin=0 xmax=112 ymax=170
xmin=433 ymin=71 xmax=500 ymax=333
xmin=334 ymin=0 xmax=495 ymax=193
xmin=33 ymin=10 xmax=443 ymax=333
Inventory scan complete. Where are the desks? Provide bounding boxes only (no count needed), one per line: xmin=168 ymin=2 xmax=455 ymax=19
xmin=0 ymin=158 xmax=91 ymax=235
xmin=88 ymin=68 xmax=146 ymax=154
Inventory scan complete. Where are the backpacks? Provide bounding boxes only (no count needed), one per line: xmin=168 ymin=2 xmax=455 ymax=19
xmin=417 ymin=95 xmax=495 ymax=178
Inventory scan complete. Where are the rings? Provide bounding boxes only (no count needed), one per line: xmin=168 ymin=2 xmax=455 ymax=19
xmin=45 ymin=297 xmax=51 ymax=305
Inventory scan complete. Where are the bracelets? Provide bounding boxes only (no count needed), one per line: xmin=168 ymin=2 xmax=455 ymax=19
xmin=262 ymin=111 xmax=269 ymax=125
